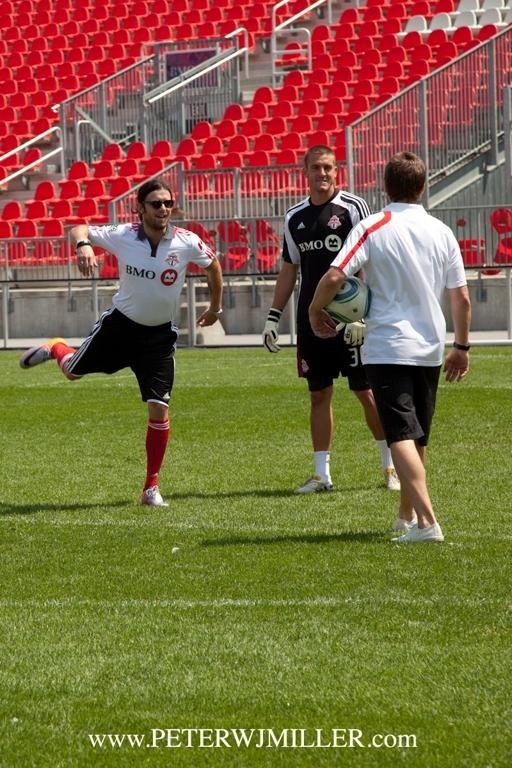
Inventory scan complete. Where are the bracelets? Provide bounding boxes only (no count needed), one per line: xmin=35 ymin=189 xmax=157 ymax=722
xmin=207 ymin=309 xmax=223 ymax=318
xmin=453 ymin=342 xmax=471 ymax=351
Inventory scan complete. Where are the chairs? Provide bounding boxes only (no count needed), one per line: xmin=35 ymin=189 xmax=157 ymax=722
xmin=1 ymin=1 xmax=511 ymax=279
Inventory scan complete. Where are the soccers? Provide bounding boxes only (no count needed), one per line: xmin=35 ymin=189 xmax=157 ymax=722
xmin=322 ymin=276 xmax=371 ymax=324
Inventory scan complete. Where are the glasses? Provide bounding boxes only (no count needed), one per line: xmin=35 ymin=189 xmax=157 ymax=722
xmin=142 ymin=200 xmax=175 ymax=209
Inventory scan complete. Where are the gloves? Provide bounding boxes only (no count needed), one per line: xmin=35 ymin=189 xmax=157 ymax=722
xmin=334 ymin=318 xmax=367 ymax=346
xmin=262 ymin=306 xmax=284 ymax=354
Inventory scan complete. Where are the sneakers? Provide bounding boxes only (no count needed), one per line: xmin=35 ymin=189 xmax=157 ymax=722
xmin=293 ymin=473 xmax=335 ymax=494
xmin=19 ymin=336 xmax=70 ymax=370
xmin=390 ymin=522 xmax=445 ymax=543
xmin=383 ymin=466 xmax=401 ymax=492
xmin=392 ymin=515 xmax=419 ymax=531
xmin=140 ymin=485 xmax=170 ymax=508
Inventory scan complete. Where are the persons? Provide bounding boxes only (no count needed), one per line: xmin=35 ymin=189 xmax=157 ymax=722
xmin=73 ymin=239 xmax=93 ymax=254
xmin=263 ymin=145 xmax=404 ymax=494
xmin=307 ymin=152 xmax=471 ymax=542
xmin=17 ymin=179 xmax=224 ymax=508
